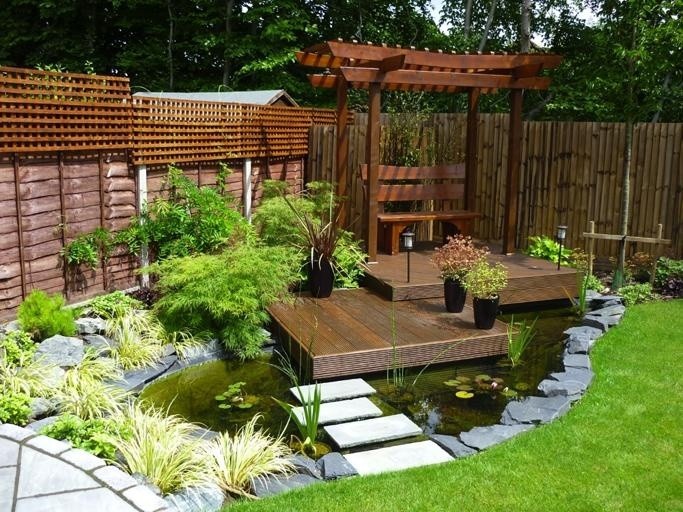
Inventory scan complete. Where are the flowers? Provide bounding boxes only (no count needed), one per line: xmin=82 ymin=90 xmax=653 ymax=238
xmin=429 ymin=229 xmax=491 ymax=283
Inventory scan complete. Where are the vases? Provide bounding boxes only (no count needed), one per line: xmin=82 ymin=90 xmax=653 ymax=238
xmin=442 ymin=277 xmax=465 ymax=312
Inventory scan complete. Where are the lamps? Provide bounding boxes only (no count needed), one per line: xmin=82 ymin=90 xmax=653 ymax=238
xmin=403 ymin=231 xmax=415 ymax=282
xmin=555 ymin=225 xmax=568 ymax=271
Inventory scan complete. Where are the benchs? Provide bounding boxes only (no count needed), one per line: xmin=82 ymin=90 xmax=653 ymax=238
xmin=359 ymin=162 xmax=484 ymax=256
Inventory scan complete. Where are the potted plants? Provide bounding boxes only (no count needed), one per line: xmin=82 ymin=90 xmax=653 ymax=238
xmin=274 ymin=185 xmax=373 ymax=299
xmin=455 ymin=256 xmax=510 ymax=330
